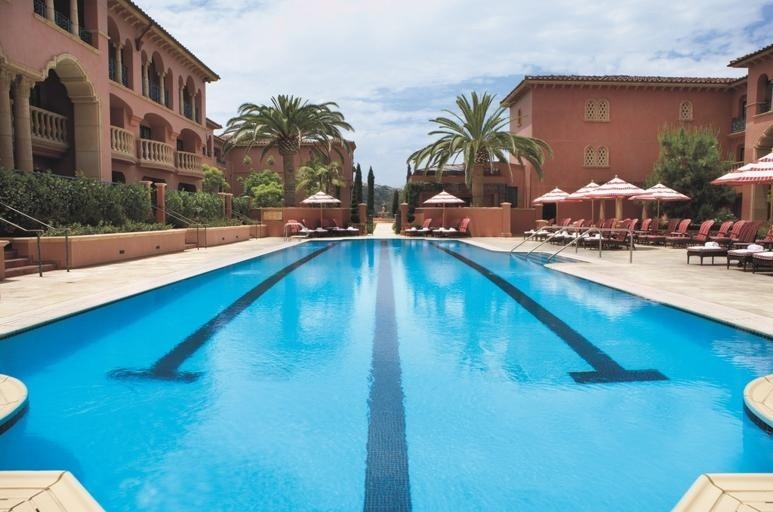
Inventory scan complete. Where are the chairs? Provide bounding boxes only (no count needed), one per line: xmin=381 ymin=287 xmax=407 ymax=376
xmin=520 ymin=215 xmax=773 ymax=276
xmin=404 ymin=217 xmax=471 ymax=237
xmin=283 ymin=215 xmax=359 ymax=236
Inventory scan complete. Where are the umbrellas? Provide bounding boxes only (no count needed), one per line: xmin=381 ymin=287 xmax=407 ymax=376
xmin=709 ymin=148 xmax=773 ymax=222
xmin=299 ymin=191 xmax=341 ymax=228
xmin=423 ymin=188 xmax=466 ymax=228
xmin=531 ymin=174 xmax=692 ymax=225
